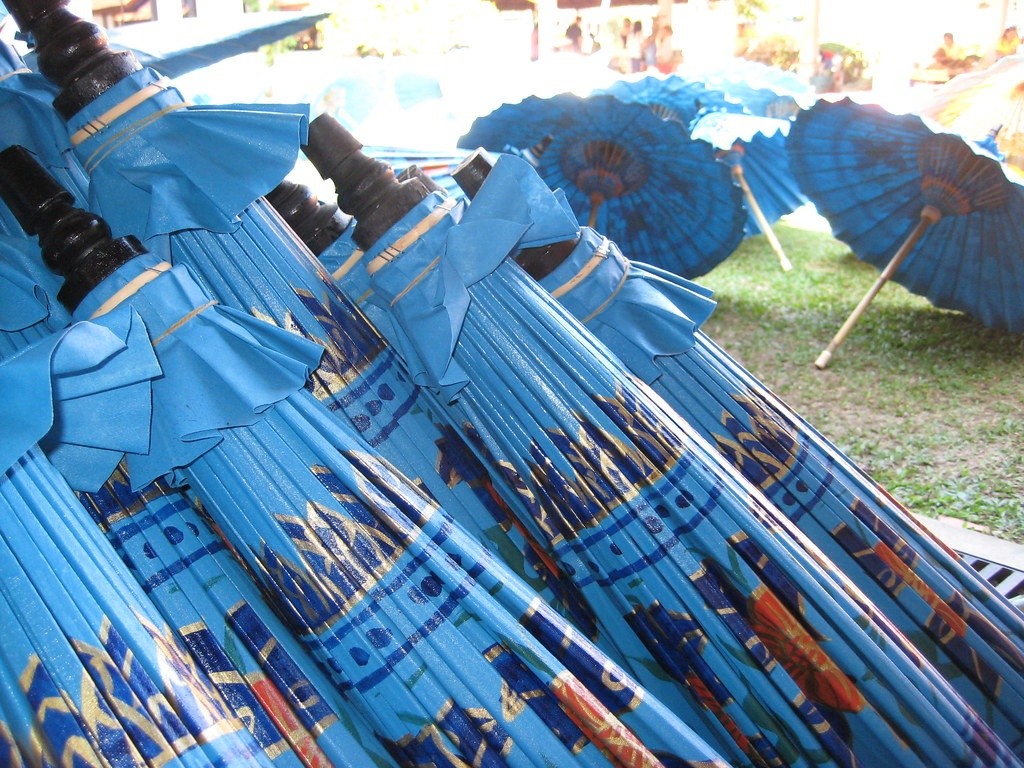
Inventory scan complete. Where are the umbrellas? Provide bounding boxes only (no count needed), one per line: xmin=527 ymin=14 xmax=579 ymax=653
xmin=0 ymin=1 xmax=1024 ymax=768
xmin=458 ymin=95 xmax=748 ymax=280
xmin=786 ymin=94 xmax=1024 ymax=369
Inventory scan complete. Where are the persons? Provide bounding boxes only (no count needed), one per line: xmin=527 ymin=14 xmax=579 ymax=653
xmin=565 ymin=16 xmax=583 ymax=42
xmin=654 ymin=27 xmax=676 ymax=74
xmin=626 ymin=21 xmax=644 ymax=74
